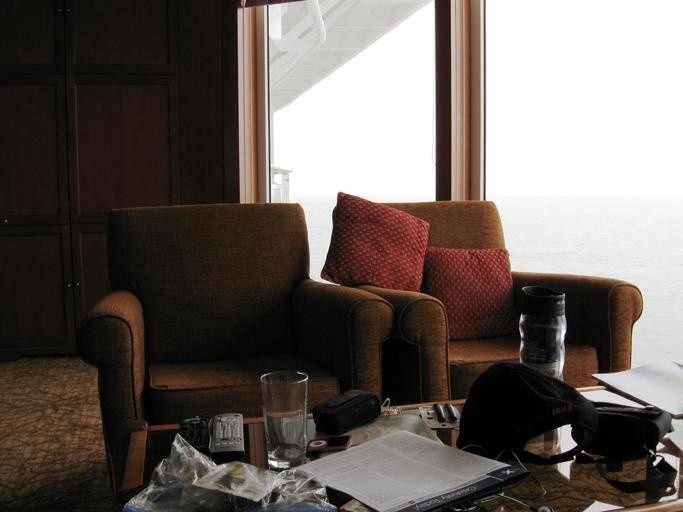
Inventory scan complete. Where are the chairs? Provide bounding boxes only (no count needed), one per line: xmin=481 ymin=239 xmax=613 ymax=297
xmin=77 ymin=204 xmax=396 ymax=452
xmin=334 ymin=203 xmax=641 ymax=452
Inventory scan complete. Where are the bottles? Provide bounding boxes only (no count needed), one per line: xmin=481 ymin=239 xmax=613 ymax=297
xmin=519 ymin=285 xmax=568 ymax=456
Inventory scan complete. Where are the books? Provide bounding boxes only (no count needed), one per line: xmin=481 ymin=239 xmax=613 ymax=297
xmin=591 ymin=361 xmax=683 ymax=418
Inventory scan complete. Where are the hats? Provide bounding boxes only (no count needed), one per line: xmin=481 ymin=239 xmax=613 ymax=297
xmin=453 ymin=358 xmax=603 ymax=465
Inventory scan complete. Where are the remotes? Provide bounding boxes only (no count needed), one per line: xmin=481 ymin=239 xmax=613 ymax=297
xmin=181 ymin=417 xmax=213 ymax=455
xmin=211 ymin=413 xmax=246 ymax=455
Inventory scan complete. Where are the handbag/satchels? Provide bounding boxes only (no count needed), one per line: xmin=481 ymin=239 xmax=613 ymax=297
xmin=570 ymin=400 xmax=675 ymax=464
xmin=310 ymin=388 xmax=383 ymax=437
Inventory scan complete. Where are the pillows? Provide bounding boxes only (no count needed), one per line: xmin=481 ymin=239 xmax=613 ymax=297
xmin=318 ymin=188 xmax=430 ymax=292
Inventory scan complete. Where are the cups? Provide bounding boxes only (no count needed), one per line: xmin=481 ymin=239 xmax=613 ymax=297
xmin=260 ymin=372 xmax=309 ymax=469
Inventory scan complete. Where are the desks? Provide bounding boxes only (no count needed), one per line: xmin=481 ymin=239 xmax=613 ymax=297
xmin=119 ymin=384 xmax=682 ymax=512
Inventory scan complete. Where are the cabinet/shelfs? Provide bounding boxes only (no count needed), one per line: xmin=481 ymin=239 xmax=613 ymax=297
xmin=0 ymin=1 xmax=238 ymax=357
